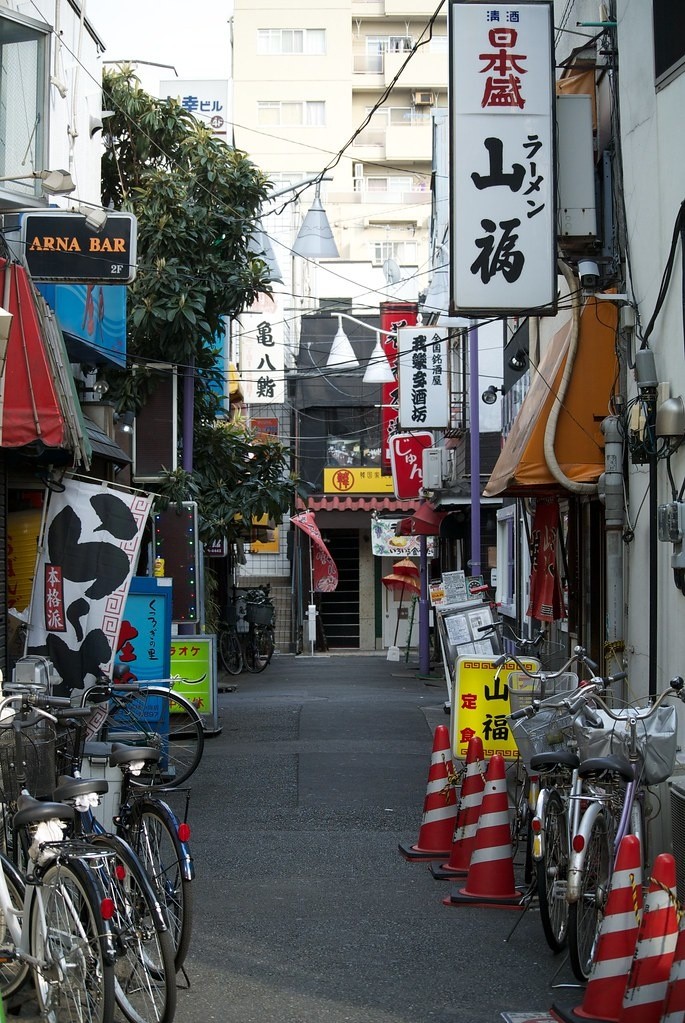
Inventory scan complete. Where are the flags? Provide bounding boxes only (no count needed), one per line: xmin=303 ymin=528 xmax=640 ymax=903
xmin=26 ymin=478 xmax=156 ymax=745
xmin=291 ymin=513 xmax=338 ymax=594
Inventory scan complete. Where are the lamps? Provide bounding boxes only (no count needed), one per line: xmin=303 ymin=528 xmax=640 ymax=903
xmin=329 ymin=312 xmax=399 ymax=384
xmin=507 ymin=345 xmax=529 ymax=372
xmin=1 ymin=206 xmax=109 ymax=234
xmin=362 ymin=330 xmax=399 ymax=383
xmin=240 ymin=207 xmax=283 ymax=282
xmin=94 ymin=367 xmax=110 ymax=393
xmin=257 ymin=177 xmax=339 ymax=256
xmin=481 ymin=384 xmax=506 ymax=404
xmin=0 ymin=170 xmax=76 ymax=196
xmin=113 ymin=411 xmax=137 ymax=435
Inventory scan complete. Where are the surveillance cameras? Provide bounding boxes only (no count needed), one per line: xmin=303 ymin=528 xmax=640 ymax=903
xmin=577 ymin=259 xmax=601 ymax=291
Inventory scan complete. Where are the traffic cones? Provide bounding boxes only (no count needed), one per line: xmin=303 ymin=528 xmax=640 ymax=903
xmin=549 ymin=835 xmax=643 ymax=1023
xmin=398 ymin=725 xmax=459 ymax=862
xmin=621 ymin=854 xmax=679 ymax=1023
xmin=659 ymin=914 xmax=685 ymax=1023
xmin=443 ymin=755 xmax=526 ymax=909
xmin=426 ymin=736 xmax=486 ymax=881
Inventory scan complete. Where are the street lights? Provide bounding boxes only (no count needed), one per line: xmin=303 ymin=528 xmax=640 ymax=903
xmin=325 ymin=312 xmax=429 ymax=674
xmin=423 ymin=242 xmax=481 ymax=578
xmin=184 ymin=175 xmax=340 ymax=473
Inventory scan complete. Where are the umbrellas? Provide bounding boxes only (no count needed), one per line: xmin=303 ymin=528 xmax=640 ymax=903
xmin=381 ymin=557 xmax=421 ymax=646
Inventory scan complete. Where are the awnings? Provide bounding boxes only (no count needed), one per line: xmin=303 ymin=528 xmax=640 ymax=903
xmin=395 ymin=504 xmax=462 ymax=540
xmin=0 ymin=257 xmax=132 ymax=476
xmin=482 ymin=288 xmax=619 ymax=497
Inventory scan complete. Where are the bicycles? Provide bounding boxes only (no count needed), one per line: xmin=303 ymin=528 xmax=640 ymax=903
xmin=478 ymin=621 xmax=566 ymax=860
xmin=502 ymin=672 xmax=628 ymax=954
xmin=491 ymin=646 xmax=598 ymax=884
xmin=0 ymin=663 xmax=207 ymax=1023
xmin=548 ymin=676 xmax=684 ymax=988
xmin=239 ymin=595 xmax=275 ymax=673
xmin=218 ymin=593 xmax=246 ymax=674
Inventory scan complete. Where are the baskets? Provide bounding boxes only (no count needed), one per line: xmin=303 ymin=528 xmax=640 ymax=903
xmin=246 ymin=601 xmax=273 ymax=625
xmin=521 ymin=641 xmax=566 ymax=671
xmin=55 ymin=730 xmax=76 ymax=782
xmin=505 ymin=689 xmax=584 ymax=776
xmin=0 ymin=727 xmax=54 ymax=796
xmin=509 ymin=671 xmax=579 ymax=711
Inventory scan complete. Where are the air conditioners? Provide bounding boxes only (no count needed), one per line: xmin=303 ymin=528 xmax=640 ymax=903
xmin=414 ymin=91 xmax=434 ymax=106
xmin=422 ymin=447 xmax=450 ymax=490
xmin=556 ymin=94 xmax=597 ymax=276
